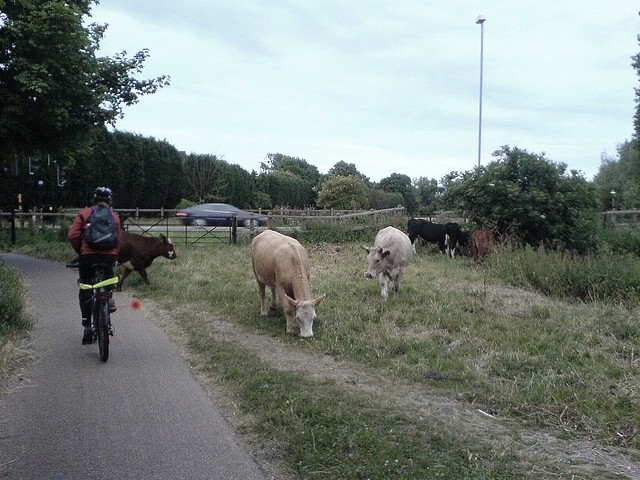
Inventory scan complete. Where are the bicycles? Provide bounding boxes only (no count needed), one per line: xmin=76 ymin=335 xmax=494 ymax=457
xmin=63 ymin=260 xmax=133 ymax=362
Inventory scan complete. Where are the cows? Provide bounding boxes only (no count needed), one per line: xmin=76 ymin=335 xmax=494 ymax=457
xmin=470 ymin=229 xmax=503 ymax=262
xmin=249 ymin=229 xmax=327 ymax=338
xmin=408 ymin=218 xmax=443 ymax=255
xmin=360 ymin=227 xmax=413 ymax=301
xmin=444 ymin=222 xmax=470 ymax=259
xmin=115 ymin=232 xmax=178 ymax=292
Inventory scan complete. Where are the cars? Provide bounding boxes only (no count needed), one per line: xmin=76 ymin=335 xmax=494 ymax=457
xmin=177 ymin=202 xmax=268 ymax=227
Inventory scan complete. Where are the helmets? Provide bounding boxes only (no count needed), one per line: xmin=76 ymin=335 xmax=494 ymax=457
xmin=93 ymin=186 xmax=113 ymax=204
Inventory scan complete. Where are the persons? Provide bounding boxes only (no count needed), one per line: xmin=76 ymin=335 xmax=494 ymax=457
xmin=67 ymin=186 xmax=122 ymax=343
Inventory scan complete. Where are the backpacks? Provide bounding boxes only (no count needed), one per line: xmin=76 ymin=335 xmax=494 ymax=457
xmin=84 ymin=205 xmax=118 ymax=251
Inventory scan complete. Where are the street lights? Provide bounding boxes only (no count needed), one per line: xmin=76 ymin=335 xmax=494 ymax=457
xmin=476 ymin=13 xmax=483 ymax=167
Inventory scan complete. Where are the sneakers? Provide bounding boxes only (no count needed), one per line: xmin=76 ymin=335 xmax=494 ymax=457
xmin=108 ymin=297 xmax=116 ymax=313
xmin=81 ymin=328 xmax=94 ymax=345
